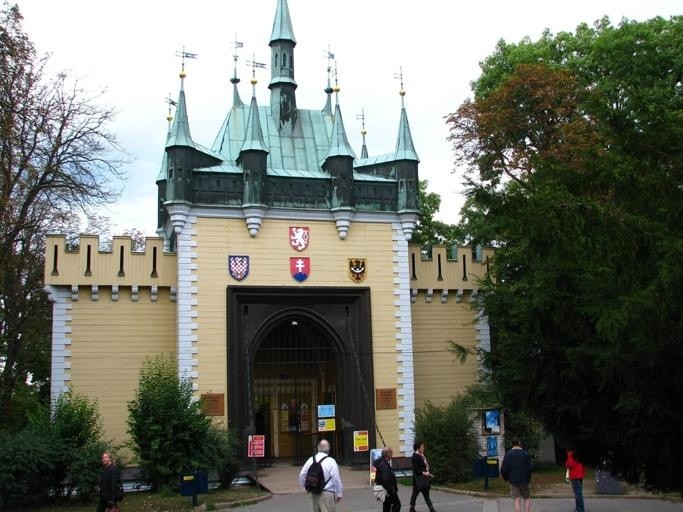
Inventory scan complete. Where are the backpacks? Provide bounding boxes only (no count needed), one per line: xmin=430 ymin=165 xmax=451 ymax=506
xmin=305 ymin=455 xmax=332 ymax=494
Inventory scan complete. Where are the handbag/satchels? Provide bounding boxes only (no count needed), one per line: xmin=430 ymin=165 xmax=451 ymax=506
xmin=565 ymin=469 xmax=570 ymax=484
xmin=414 ymin=474 xmax=431 ymax=491
xmin=114 ymin=481 xmax=123 ymax=502
xmin=374 ymin=484 xmax=388 ymax=501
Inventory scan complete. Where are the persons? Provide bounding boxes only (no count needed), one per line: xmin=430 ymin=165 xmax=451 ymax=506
xmin=299 ymin=440 xmax=344 ymax=512
xmin=500 ymin=437 xmax=535 ymax=512
xmin=565 ymin=437 xmax=586 ymax=512
xmin=373 ymin=447 xmax=401 ymax=512
xmin=255 ymin=397 xmax=300 ymax=435
xmin=409 ymin=440 xmax=436 ymax=512
xmin=95 ymin=452 xmax=119 ymax=512
